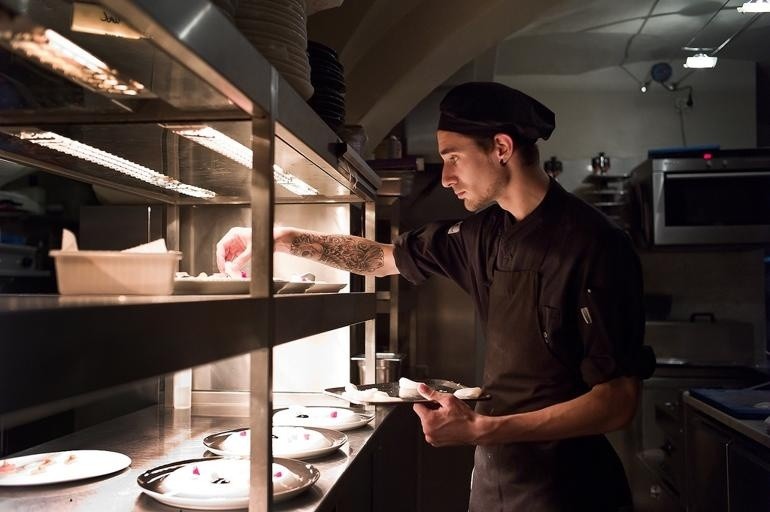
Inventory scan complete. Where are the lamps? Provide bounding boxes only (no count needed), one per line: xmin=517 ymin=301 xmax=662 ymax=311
xmin=737 ymin=0 xmax=770 ymax=13
xmin=680 ymin=45 xmax=717 ymax=69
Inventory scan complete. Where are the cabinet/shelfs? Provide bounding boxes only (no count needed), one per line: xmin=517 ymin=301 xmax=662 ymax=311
xmin=583 ymin=173 xmax=631 ymax=237
xmin=0 ymin=0 xmax=425 ymax=512
xmin=682 ymin=391 xmax=770 ymax=512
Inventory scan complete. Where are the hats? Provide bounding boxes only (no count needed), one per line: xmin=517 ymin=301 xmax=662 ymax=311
xmin=437 ymin=79 xmax=556 ymax=142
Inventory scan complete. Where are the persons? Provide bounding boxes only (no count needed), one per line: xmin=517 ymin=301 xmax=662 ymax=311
xmin=217 ymin=82 xmax=656 ymax=511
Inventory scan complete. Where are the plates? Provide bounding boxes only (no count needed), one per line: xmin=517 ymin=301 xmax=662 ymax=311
xmin=174 ymin=276 xmax=346 ymax=294
xmin=135 ymin=457 xmax=320 ymax=509
xmin=223 ymin=0 xmax=347 ymax=133
xmin=270 ymin=405 xmax=375 ymax=433
xmin=203 ymin=427 xmax=350 ymax=462
xmin=0 ymin=449 xmax=131 ymax=486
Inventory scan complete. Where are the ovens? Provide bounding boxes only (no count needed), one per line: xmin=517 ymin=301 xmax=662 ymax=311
xmin=626 ymin=154 xmax=769 ymax=247
